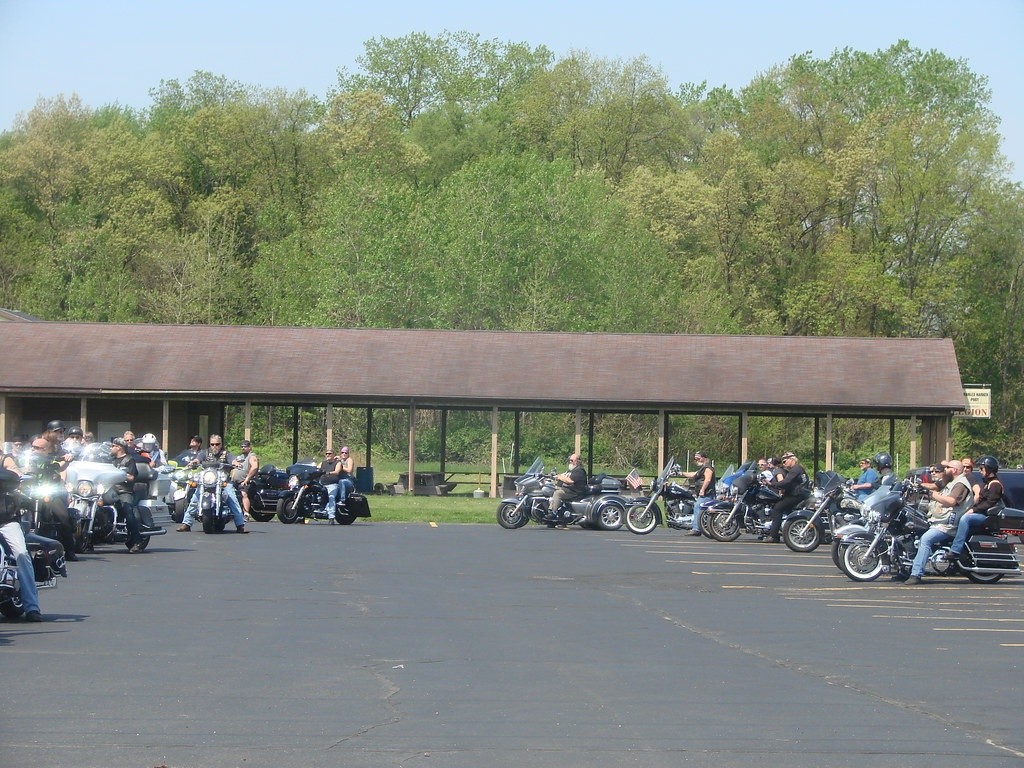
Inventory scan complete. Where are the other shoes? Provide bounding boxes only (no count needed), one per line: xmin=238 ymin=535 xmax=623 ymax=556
xmin=176 ymin=524 xmax=191 ymax=532
xmin=336 ymin=500 xmax=346 ymax=506
xmin=65 ymin=552 xmax=80 ymax=561
xmin=761 ymin=536 xmax=779 ymax=543
xmin=236 ymin=514 xmax=250 ymax=533
xmin=892 ymin=572 xmax=921 ymax=585
xmin=26 ymin=610 xmax=44 ymax=622
xmin=544 ymin=512 xmax=560 ymax=520
xmin=329 ymin=518 xmax=334 ymax=525
xmin=942 ymin=551 xmax=958 ymax=559
xmin=684 ymin=530 xmax=701 ymax=536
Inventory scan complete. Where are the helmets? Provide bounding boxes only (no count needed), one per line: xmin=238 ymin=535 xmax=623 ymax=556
xmin=873 ymin=452 xmax=893 ymax=472
xmin=45 ymin=421 xmax=84 ymax=437
xmin=114 ymin=433 xmax=156 ymax=447
xmin=975 ymin=455 xmax=999 ymax=475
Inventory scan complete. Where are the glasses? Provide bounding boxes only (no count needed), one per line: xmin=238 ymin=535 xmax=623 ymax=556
xmin=210 ymin=443 xmax=249 ymax=449
xmin=694 ymin=457 xmax=701 ymax=461
xmin=928 ymin=465 xmax=983 ymax=474
xmin=568 ymin=459 xmax=575 ymax=462
xmin=12 ymin=429 xmax=80 ymax=451
xmin=757 ymin=463 xmax=767 ymax=466
xmin=111 ymin=438 xmax=135 ymax=448
xmin=326 ymin=452 xmax=348 ymax=455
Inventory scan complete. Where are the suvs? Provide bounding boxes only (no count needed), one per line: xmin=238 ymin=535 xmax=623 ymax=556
xmin=905 ymin=468 xmax=1024 ymax=512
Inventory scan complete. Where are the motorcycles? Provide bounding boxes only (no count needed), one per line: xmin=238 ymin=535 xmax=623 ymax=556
xmin=0 ymin=435 xmax=372 ymax=623
xmin=495 ymin=456 xmax=1024 ymax=586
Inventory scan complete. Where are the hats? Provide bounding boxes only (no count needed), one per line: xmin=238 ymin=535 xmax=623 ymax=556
xmin=326 ymin=446 xmax=349 ymax=454
xmin=192 ymin=435 xmax=202 ymax=443
xmin=940 ymin=457 xmax=972 ymax=469
xmin=857 ymin=458 xmax=871 ymax=464
xmin=695 ymin=450 xmax=708 ymax=458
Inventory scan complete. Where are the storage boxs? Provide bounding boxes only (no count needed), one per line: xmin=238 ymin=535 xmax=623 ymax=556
xmin=138 ymin=499 xmax=173 ymax=527
xmin=997 ymin=507 xmax=1024 ymax=530
xmin=968 ymin=535 xmax=1018 ymax=568
xmin=149 ymin=474 xmax=171 ymax=496
xmin=256 ymin=489 xmax=280 ymax=509
xmin=25 ymin=533 xmax=68 ymax=582
xmin=347 ymin=494 xmax=370 ymax=516
xmin=601 ymin=474 xmax=622 ymax=491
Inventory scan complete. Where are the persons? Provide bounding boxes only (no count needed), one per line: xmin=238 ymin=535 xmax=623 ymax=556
xmin=315 ymin=447 xmax=357 ymax=525
xmin=848 ymin=451 xmax=1005 ymax=586
xmin=544 ymin=454 xmax=588 ymax=519
xmin=748 ymin=451 xmax=811 ymax=544
xmin=673 ymin=450 xmax=717 ymax=536
xmin=0 ymin=419 xmax=259 ymax=622
